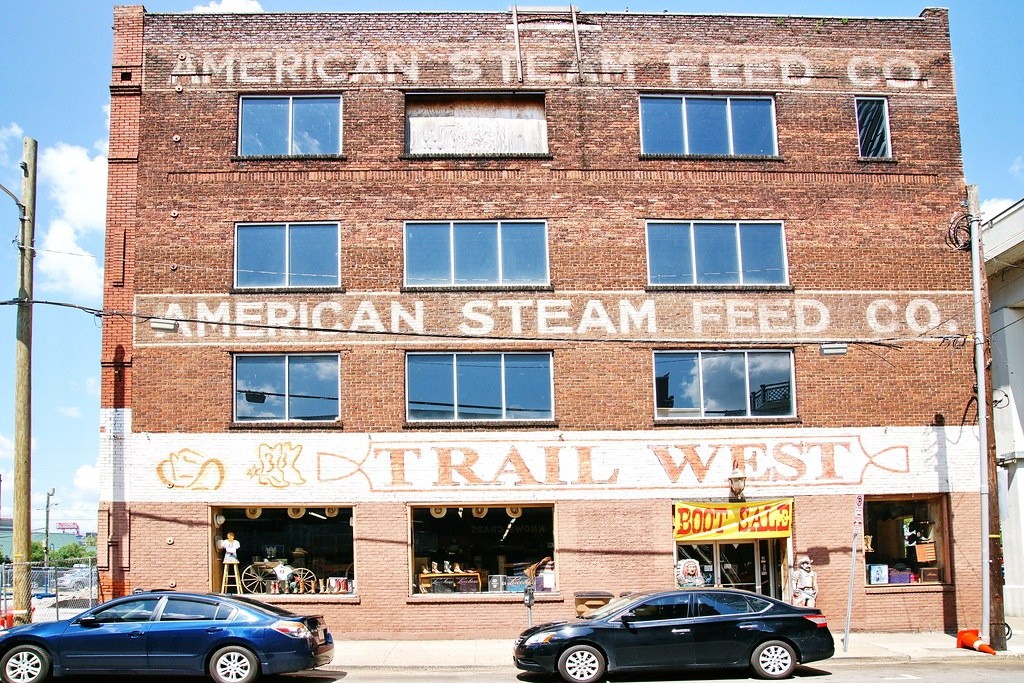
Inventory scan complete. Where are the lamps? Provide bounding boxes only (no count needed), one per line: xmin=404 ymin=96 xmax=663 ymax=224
xmin=150 ymin=318 xmax=180 ymax=334
xmin=725 ymin=458 xmax=746 ymax=502
xmin=819 ymin=343 xmax=848 ymax=356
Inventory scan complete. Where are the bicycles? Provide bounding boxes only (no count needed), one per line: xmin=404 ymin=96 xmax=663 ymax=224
xmin=241 ymin=553 xmax=318 ymax=594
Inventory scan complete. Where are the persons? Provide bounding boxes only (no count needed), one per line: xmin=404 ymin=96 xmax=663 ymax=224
xmin=791 ymin=555 xmax=818 ymax=608
xmin=677 ymin=559 xmax=705 ymax=588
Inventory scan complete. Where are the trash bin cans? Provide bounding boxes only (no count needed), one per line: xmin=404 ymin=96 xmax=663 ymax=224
xmin=573 ymin=591 xmax=615 ymax=620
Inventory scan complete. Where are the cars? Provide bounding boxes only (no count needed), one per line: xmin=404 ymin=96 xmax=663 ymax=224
xmin=0 ymin=588 xmax=334 ymax=683
xmin=0 ymin=563 xmax=97 ymax=601
xmin=513 ymin=586 xmax=834 ymax=683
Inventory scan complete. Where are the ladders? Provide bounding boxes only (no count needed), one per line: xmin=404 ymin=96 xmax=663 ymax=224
xmin=221 ymin=562 xmax=243 ymax=594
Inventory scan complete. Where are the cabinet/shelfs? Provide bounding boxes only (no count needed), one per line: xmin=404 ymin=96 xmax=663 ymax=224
xmin=876 ymin=514 xmax=914 ymax=560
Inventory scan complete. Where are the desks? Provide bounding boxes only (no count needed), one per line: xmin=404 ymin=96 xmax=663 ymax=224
xmin=919 ymin=567 xmax=944 ymax=583
xmin=419 ymin=573 xmax=482 ymax=593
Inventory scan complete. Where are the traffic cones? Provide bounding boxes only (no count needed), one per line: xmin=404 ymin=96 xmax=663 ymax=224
xmin=955 ymin=629 xmax=997 ymax=656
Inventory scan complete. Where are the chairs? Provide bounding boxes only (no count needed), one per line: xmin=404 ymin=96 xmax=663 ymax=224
xmin=675 ymin=602 xmax=687 ymax=618
